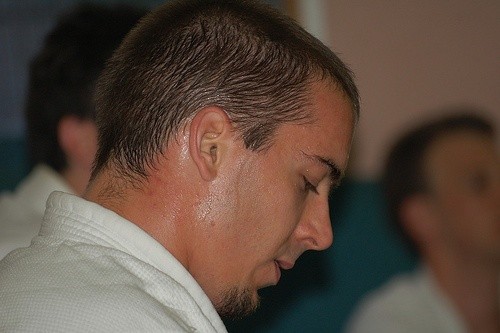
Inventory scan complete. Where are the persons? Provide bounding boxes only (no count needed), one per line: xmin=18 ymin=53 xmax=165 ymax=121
xmin=345 ymin=114 xmax=500 ymax=333
xmin=0 ymin=1 xmax=360 ymax=333
